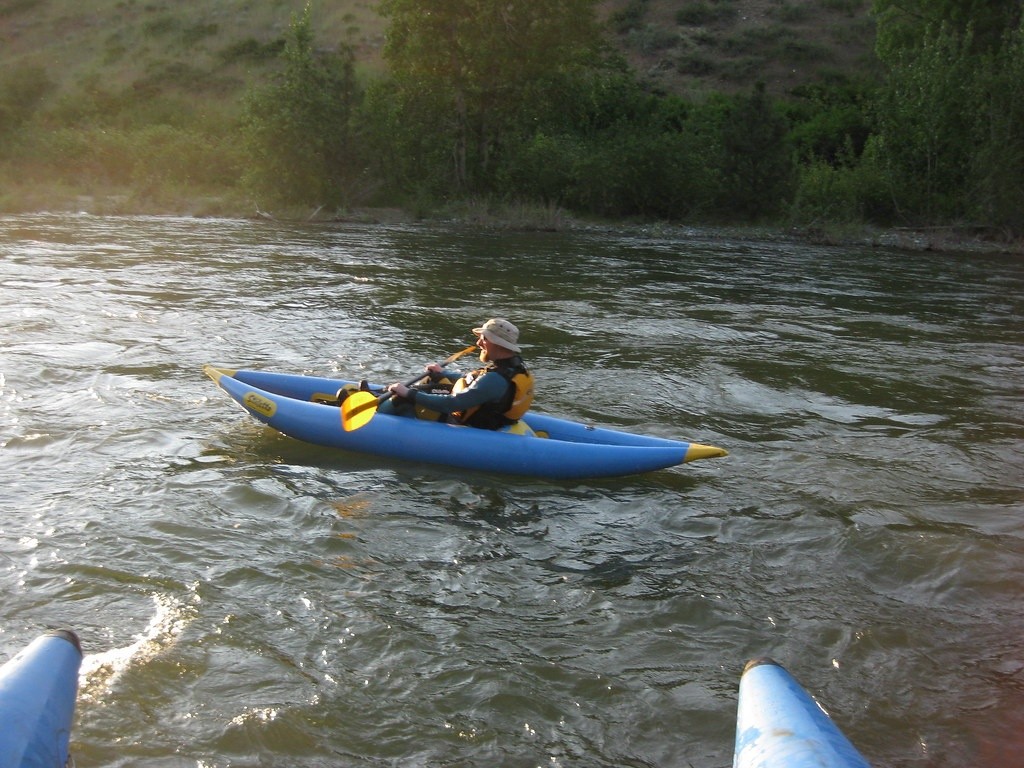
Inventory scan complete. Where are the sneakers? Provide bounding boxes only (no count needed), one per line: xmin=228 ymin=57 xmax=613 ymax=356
xmin=361 ymin=380 xmax=374 ymax=397
xmin=336 ymin=388 xmax=349 ymax=407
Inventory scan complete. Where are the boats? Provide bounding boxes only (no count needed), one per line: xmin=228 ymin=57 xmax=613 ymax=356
xmin=197 ymin=365 xmax=729 ymax=479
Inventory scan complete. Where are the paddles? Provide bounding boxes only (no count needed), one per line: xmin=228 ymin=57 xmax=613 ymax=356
xmin=341 ymin=345 xmax=476 ymax=432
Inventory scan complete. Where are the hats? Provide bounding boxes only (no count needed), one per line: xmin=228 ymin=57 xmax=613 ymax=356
xmin=472 ymin=319 xmax=521 ymax=354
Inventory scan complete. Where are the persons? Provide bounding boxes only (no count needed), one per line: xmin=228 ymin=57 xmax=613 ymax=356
xmin=335 ymin=315 xmax=533 ymax=431
